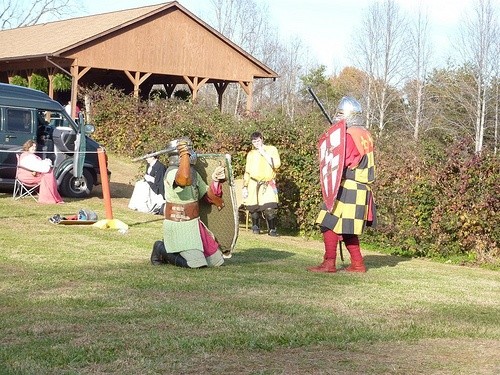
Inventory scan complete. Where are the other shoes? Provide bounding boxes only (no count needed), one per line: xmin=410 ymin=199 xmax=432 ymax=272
xmin=306 ymin=259 xmax=337 ymax=273
xmin=344 ymin=260 xmax=366 ymax=272
xmin=268 ymin=229 xmax=277 ymax=236
xmin=253 ymin=228 xmax=260 ymax=234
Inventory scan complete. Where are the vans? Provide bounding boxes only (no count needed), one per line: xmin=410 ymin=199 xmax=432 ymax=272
xmin=0 ymin=83 xmax=111 ymax=199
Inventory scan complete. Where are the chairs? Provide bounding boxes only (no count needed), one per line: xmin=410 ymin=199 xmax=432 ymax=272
xmin=234 ymin=180 xmax=271 ymax=232
xmin=13 ymin=154 xmax=41 ymax=203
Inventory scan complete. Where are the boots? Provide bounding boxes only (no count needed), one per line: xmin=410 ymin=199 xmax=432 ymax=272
xmin=151 ymin=240 xmax=180 ymax=265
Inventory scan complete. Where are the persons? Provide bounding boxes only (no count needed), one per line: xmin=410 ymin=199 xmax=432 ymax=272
xmin=128 ymin=150 xmax=167 ymax=214
xmin=242 ymin=132 xmax=281 ymax=237
xmin=18 ymin=139 xmax=65 ymax=204
xmin=306 ymin=96 xmax=378 ymax=274
xmin=151 ymin=137 xmax=225 ymax=269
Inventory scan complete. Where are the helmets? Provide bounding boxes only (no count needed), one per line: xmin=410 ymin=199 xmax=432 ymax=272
xmin=166 ymin=136 xmax=197 ymax=166
xmin=333 ymin=97 xmax=363 ymax=125
